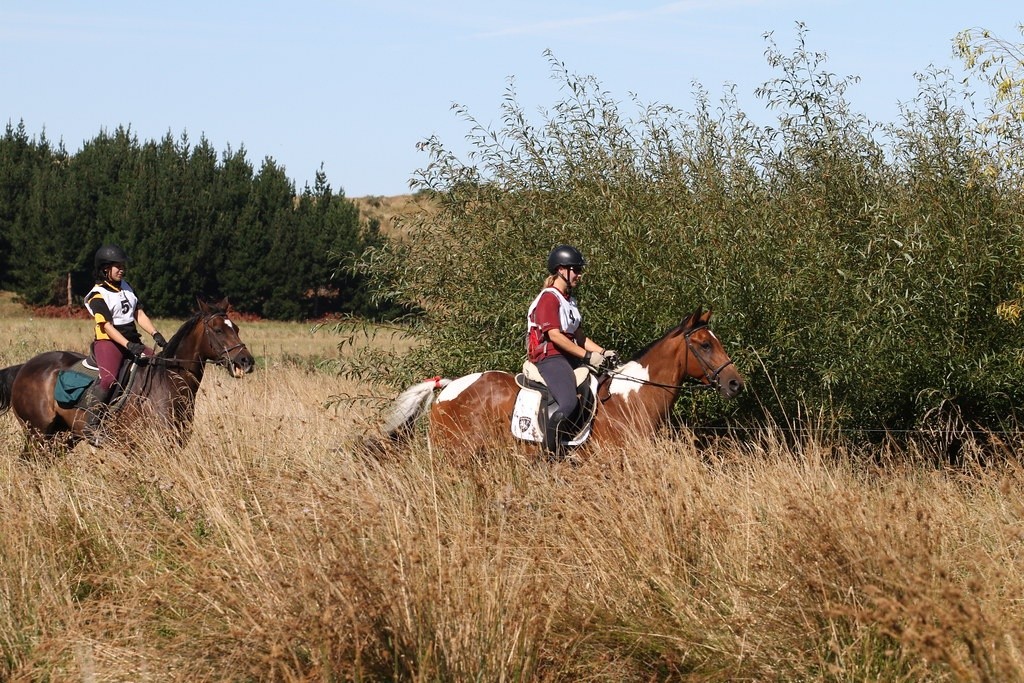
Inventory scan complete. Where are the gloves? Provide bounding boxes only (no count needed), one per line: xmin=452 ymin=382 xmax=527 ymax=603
xmin=604 ymin=350 xmax=616 ymax=358
xmin=153 ymin=332 xmax=167 ymax=346
xmin=127 ymin=342 xmax=145 ymax=356
xmin=590 ymin=352 xmax=606 ymax=369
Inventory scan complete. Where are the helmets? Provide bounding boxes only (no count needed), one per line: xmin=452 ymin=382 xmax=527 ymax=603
xmin=95 ymin=246 xmax=128 ymax=279
xmin=548 ymin=246 xmax=587 ymax=273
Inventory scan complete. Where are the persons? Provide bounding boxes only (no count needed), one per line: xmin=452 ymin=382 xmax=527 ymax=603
xmin=525 ymin=244 xmax=622 ymax=463
xmin=80 ymin=244 xmax=166 ymax=448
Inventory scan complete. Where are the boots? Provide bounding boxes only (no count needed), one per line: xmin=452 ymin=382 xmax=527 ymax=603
xmin=542 ymin=411 xmax=575 ymax=466
xmin=83 ymin=387 xmax=111 ymax=446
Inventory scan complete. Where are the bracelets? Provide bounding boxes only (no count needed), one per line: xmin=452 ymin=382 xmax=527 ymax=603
xmin=600 ymin=348 xmax=605 ymax=354
xmin=151 ymin=330 xmax=157 ymax=336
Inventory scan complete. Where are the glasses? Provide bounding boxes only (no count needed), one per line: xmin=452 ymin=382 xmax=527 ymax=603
xmin=562 ymin=266 xmax=582 ymax=273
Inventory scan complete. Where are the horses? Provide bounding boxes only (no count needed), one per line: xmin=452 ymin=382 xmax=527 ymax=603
xmin=0 ymin=295 xmax=256 ymax=465
xmin=351 ymin=303 xmax=744 ymax=469
